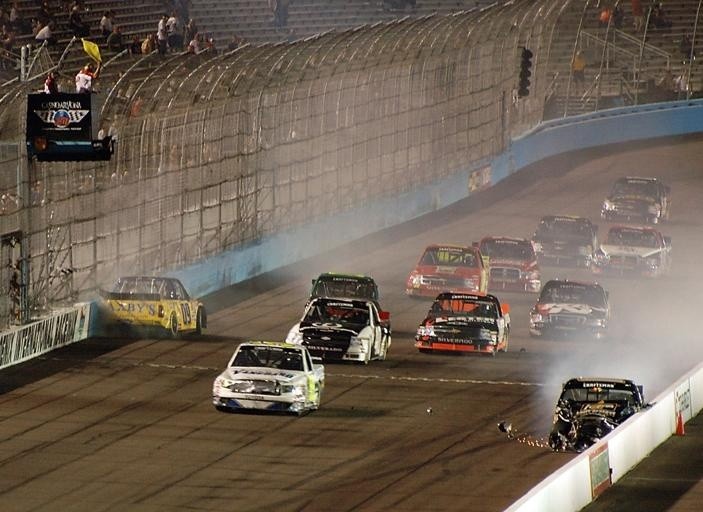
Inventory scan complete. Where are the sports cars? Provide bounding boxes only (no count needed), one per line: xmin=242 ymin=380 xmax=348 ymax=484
xmin=212 ymin=271 xmax=391 ymax=417
xmin=96 ymin=276 xmax=206 ymax=338
xmin=548 ymin=378 xmax=642 ymax=454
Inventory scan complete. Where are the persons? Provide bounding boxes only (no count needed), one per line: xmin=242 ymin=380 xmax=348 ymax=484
xmin=675 ymin=72 xmax=692 ymax=100
xmin=677 ymin=32 xmax=696 ymax=60
xmin=600 ymin=0 xmax=674 ymax=41
xmin=569 ymin=49 xmax=585 ymax=93
xmin=0 ymin=1 xmax=269 ymax=216
